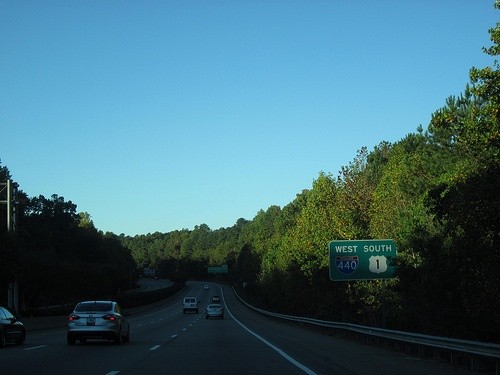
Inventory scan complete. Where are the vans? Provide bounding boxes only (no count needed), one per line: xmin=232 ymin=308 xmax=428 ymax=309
xmin=183 ymin=297 xmax=200 ymax=314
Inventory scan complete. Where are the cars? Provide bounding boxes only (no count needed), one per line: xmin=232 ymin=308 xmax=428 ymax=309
xmin=0 ymin=306 xmax=26 ymax=347
xmin=212 ymin=296 xmax=220 ymax=303
xmin=204 ymin=285 xmax=209 ymax=290
xmin=68 ymin=301 xmax=129 ymax=343
xmin=205 ymin=304 xmax=224 ymax=319
